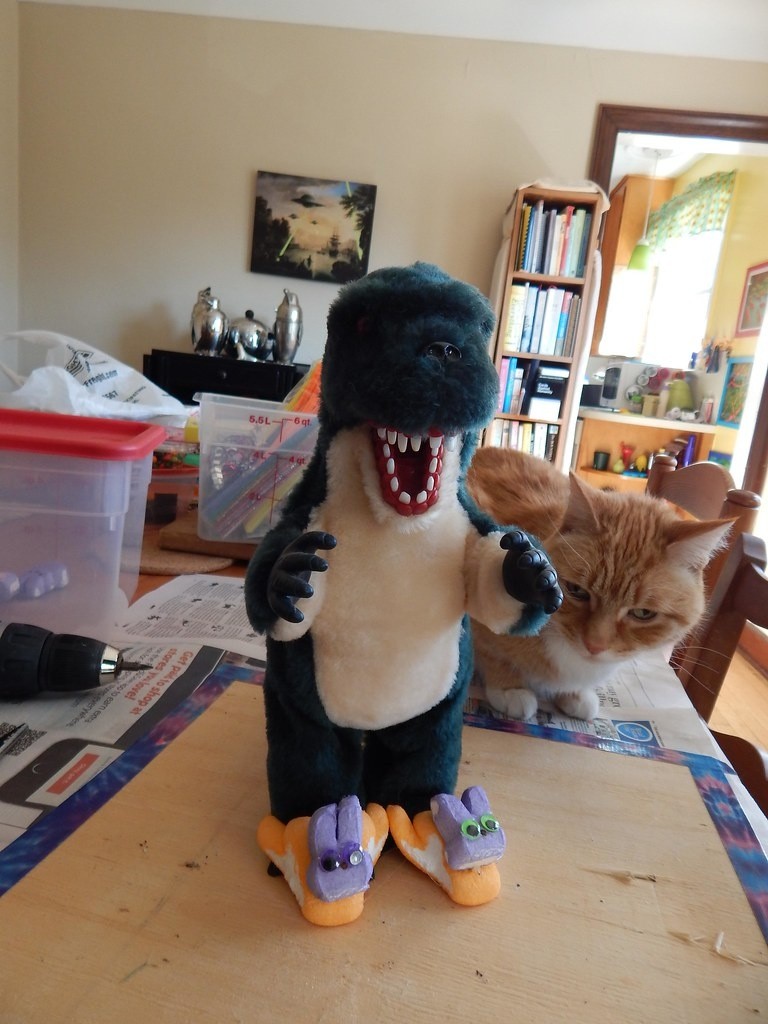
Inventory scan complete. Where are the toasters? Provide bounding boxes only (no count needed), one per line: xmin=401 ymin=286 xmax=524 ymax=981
xmin=599 ymin=361 xmax=680 ymax=413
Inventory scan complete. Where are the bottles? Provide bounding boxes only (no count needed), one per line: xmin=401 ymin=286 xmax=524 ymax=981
xmin=274 ymin=288 xmax=304 ymax=368
xmin=189 ymin=287 xmax=228 ymax=357
xmin=224 ymin=310 xmax=278 ymax=363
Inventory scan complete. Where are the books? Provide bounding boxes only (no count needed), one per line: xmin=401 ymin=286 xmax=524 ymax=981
xmin=481 ymin=199 xmax=594 ymax=462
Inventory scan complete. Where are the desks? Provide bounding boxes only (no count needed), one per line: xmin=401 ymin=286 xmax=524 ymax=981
xmin=0 ymin=522 xmax=767 ymax=1023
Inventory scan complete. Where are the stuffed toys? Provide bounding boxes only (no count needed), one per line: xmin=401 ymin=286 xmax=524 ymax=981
xmin=243 ymin=261 xmax=567 ymax=928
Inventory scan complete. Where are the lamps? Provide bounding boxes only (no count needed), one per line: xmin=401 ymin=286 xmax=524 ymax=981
xmin=628 ymin=147 xmax=668 ymax=269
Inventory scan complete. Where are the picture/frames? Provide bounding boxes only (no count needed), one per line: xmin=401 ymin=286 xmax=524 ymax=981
xmin=733 ymin=261 xmax=768 ymax=338
xmin=715 ymin=356 xmax=754 ymax=429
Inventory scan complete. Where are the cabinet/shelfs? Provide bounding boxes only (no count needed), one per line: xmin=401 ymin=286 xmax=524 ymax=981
xmin=590 ymin=174 xmax=676 ymax=359
xmin=143 ymin=348 xmax=311 ymax=406
xmin=575 ymin=407 xmax=715 ymax=496
xmin=481 ymin=187 xmax=602 ymax=472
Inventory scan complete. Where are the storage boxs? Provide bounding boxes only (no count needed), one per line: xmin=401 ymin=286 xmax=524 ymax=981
xmin=193 ymin=392 xmax=321 ymax=546
xmin=528 ymin=377 xmax=566 ymax=422
xmin=0 ymin=407 xmax=169 ymax=641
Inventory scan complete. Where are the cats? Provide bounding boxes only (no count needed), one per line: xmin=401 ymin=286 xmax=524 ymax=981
xmin=466 ymin=446 xmax=739 ymax=722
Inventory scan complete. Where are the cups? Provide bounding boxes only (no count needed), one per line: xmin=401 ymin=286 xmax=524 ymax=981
xmin=593 ymin=451 xmax=611 ymax=471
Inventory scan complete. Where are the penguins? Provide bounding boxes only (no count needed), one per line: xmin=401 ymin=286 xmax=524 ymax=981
xmin=189 ymin=285 xmax=227 ymax=357
xmin=271 ymin=289 xmax=304 ymax=366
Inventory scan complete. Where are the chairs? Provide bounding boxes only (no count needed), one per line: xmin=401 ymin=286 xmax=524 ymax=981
xmin=677 ymin=532 xmax=767 ymax=819
xmin=644 ymin=455 xmax=762 ymax=688
